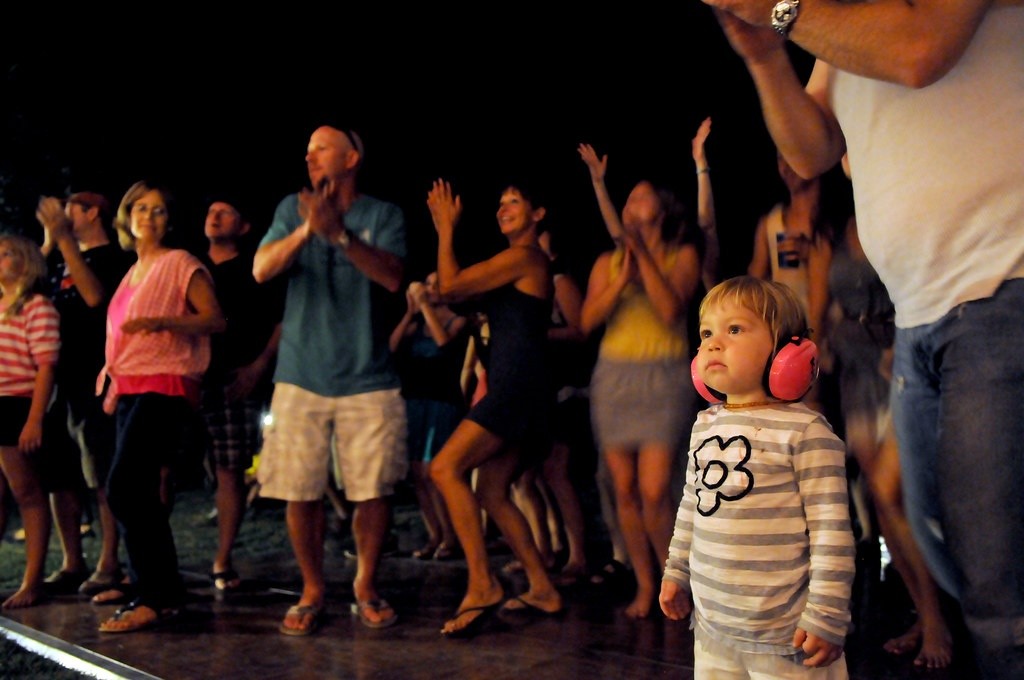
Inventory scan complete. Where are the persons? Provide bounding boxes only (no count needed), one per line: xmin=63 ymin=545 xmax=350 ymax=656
xmin=160 ymin=193 xmax=284 ymax=602
xmin=0 ymin=233 xmax=61 ymax=608
xmin=699 ymin=0 xmax=1023 ymax=653
xmin=580 ymin=179 xmax=699 ymax=619
xmin=867 ymin=340 xmax=953 ymax=671
xmin=93 ymin=181 xmax=226 ymax=633
xmin=426 ymin=177 xmax=563 ymax=635
xmin=659 ymin=276 xmax=856 ymax=680
xmin=252 ymin=125 xmax=408 ymax=636
xmin=37 ymin=192 xmax=129 ymax=595
xmin=577 ymin=118 xmax=716 ymax=273
xmin=232 ymin=220 xmax=896 ymax=588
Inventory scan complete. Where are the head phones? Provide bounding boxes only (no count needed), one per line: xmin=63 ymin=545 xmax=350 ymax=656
xmin=691 ymin=322 xmax=821 ymax=402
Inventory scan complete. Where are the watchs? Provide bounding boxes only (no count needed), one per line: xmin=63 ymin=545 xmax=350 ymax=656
xmin=771 ymin=0 xmax=799 ymax=36
xmin=333 ymin=231 xmax=355 ymax=249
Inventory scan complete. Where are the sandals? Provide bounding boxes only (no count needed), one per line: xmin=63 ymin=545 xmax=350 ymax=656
xmin=591 ymin=559 xmax=634 ymax=585
xmin=91 ymin=582 xmax=145 ymax=605
xmin=95 ymin=596 xmax=181 ymax=633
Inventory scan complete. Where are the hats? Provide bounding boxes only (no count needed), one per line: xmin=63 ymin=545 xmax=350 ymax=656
xmin=60 ymin=192 xmax=106 ymax=210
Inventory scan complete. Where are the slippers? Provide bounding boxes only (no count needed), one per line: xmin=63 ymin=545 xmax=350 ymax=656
xmin=501 ymin=594 xmax=552 ymax=622
xmin=445 ymin=594 xmax=503 ymax=637
xmin=209 ymin=565 xmax=240 ymax=592
xmin=279 ymin=601 xmax=322 ymax=636
xmin=350 ymin=598 xmax=397 ymax=629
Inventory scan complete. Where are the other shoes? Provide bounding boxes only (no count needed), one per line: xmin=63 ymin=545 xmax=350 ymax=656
xmin=411 ymin=539 xmax=437 ymax=559
xmin=80 ymin=527 xmax=96 ymax=540
xmin=4 ymin=526 xmax=25 ymax=544
xmin=434 ymin=542 xmax=462 ymax=559
xmin=504 ymin=542 xmax=590 ymax=583
xmin=198 ymin=507 xmax=218 ymax=524
xmin=44 ymin=569 xmax=89 ymax=597
xmin=78 ymin=574 xmax=122 ymax=597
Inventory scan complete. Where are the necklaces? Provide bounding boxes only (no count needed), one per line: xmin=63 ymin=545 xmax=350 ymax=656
xmin=723 ymin=401 xmax=771 ymax=408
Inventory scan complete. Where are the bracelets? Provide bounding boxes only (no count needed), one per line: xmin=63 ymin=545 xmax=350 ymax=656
xmin=301 ymin=220 xmax=315 ymax=240
